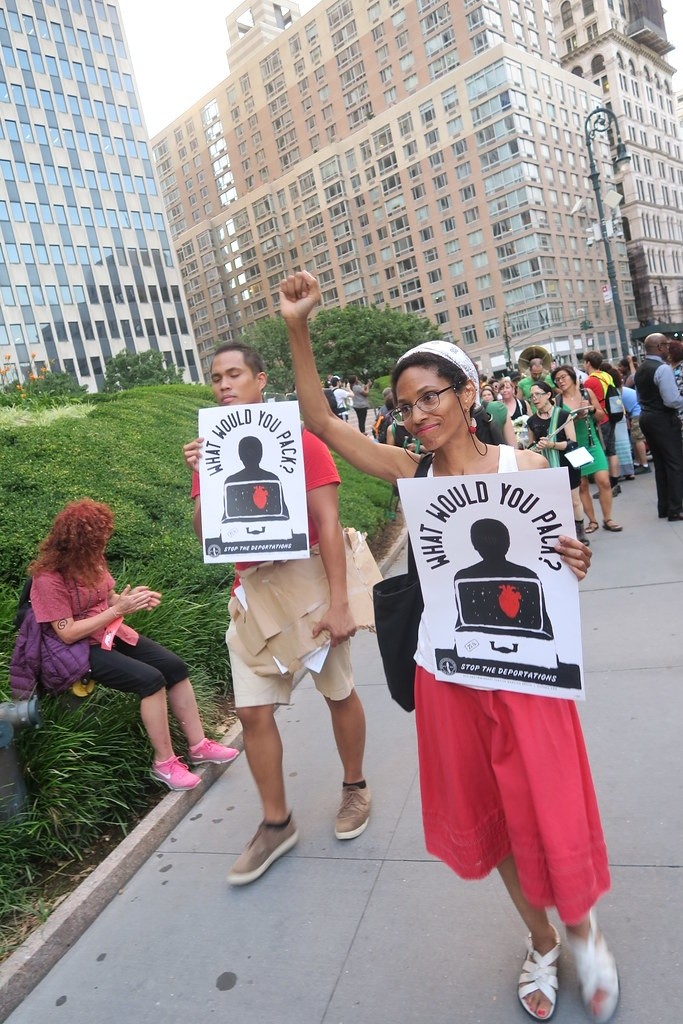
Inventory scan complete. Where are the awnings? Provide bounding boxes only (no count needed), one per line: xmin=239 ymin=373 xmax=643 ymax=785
xmin=631 ymin=323 xmax=683 ymax=340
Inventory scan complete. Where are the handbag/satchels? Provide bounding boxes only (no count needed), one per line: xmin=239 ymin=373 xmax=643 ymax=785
xmin=372 ymin=573 xmax=425 ymax=712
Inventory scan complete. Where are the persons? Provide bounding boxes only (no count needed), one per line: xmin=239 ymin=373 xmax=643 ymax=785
xmin=372 ymin=387 xmax=431 ymax=454
xmin=279 ymin=269 xmax=620 ymax=1024
xmin=288 ymin=373 xmax=372 ymax=436
xmin=182 ymin=344 xmax=371 ymax=887
xmin=634 ymin=332 xmax=683 ymax=521
xmin=514 ymin=358 xmax=623 ymax=546
xmin=478 ymin=364 xmax=533 ymax=450
xmin=581 ymin=350 xmax=650 ymax=499
xmin=32 ymin=501 xmax=240 ymax=792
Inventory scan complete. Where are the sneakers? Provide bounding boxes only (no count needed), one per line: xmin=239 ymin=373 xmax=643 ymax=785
xmin=226 ymin=812 xmax=299 ymax=885
xmin=634 ymin=464 xmax=651 ymax=474
xmin=189 ymin=738 xmax=240 ymax=764
xmin=149 ymin=755 xmax=201 ymax=790
xmin=334 ymin=783 xmax=371 ymax=839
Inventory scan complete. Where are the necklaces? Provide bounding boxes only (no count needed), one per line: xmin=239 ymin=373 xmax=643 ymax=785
xmin=70 ymin=575 xmax=92 ymax=620
xmin=537 ymin=406 xmax=552 ymax=417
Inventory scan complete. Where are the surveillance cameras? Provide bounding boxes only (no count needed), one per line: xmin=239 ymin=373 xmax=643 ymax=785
xmin=587 ymin=241 xmax=593 ymax=247
xmin=617 ymin=232 xmax=623 ymax=239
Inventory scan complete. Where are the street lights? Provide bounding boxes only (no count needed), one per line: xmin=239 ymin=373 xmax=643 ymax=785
xmin=582 ymin=105 xmax=634 ymax=380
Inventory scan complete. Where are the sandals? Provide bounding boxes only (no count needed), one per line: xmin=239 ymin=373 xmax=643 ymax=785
xmin=567 ymin=912 xmax=621 ymax=1024
xmin=602 ymin=518 xmax=622 ymax=532
xmin=518 ymin=923 xmax=562 ymax=1022
xmin=585 ymin=521 xmax=598 ymax=533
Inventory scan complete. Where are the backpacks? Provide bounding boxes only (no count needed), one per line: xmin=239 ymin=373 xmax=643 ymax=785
xmin=15 ymin=565 xmax=72 ymax=632
xmin=372 ymin=416 xmax=392 ymax=444
xmin=323 ymin=387 xmax=349 ymax=413
xmin=588 ymin=372 xmax=625 ymax=422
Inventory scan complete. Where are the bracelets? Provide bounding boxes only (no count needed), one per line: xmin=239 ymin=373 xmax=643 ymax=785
xmin=592 ymin=408 xmax=596 ymax=414
xmin=550 ymin=442 xmax=555 ymax=449
xmin=109 ymin=607 xmax=119 ymax=620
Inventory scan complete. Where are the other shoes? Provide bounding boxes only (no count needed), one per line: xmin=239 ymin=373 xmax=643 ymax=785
xmin=624 ymin=474 xmax=635 ymax=480
xmin=668 ymin=510 xmax=683 ymax=521
xmin=612 ymin=485 xmax=621 ymax=497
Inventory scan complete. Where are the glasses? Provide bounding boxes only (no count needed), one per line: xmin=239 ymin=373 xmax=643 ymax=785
xmin=554 ymin=374 xmax=571 ymax=383
xmin=658 ymin=342 xmax=672 ymax=349
xmin=389 ymin=380 xmax=465 ymax=423
xmin=530 ymin=392 xmax=547 ymax=400
